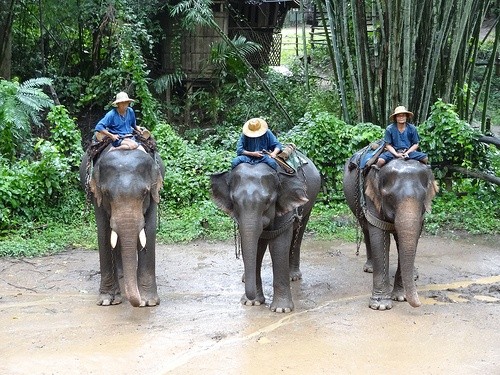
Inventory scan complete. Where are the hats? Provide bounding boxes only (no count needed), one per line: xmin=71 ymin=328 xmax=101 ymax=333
xmin=111 ymin=92 xmax=135 ymax=107
xmin=243 ymin=118 xmax=268 ymax=138
xmin=389 ymin=106 xmax=414 ymax=121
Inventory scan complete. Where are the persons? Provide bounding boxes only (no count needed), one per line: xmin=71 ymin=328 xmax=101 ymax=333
xmin=376 ymin=106 xmax=428 ymax=167
xmin=94 ymin=92 xmax=143 ymax=152
xmin=231 ymin=118 xmax=282 ymax=172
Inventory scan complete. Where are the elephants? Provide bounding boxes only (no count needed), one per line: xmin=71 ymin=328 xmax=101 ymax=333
xmin=209 ymin=148 xmax=322 ymax=313
xmin=343 ymin=145 xmax=440 ymax=311
xmin=79 ymin=137 xmax=165 ymax=308
